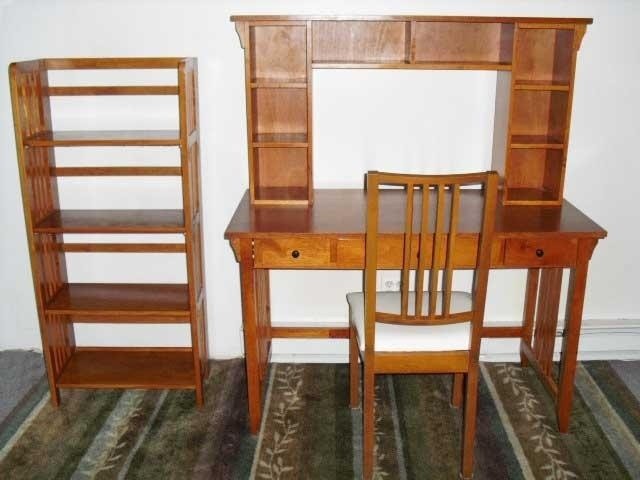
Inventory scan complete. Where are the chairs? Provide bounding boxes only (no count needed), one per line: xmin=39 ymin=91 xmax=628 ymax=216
xmin=345 ymin=170 xmax=500 ymax=479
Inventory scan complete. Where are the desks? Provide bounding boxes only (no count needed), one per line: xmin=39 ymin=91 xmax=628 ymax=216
xmin=223 ymin=190 xmax=607 ymax=438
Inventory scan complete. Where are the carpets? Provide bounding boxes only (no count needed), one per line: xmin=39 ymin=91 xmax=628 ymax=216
xmin=0 ymin=362 xmax=639 ymax=476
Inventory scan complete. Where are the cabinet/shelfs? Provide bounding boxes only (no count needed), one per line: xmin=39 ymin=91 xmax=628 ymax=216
xmin=8 ymin=56 xmax=211 ymax=412
xmin=230 ymin=13 xmax=592 ymax=206
